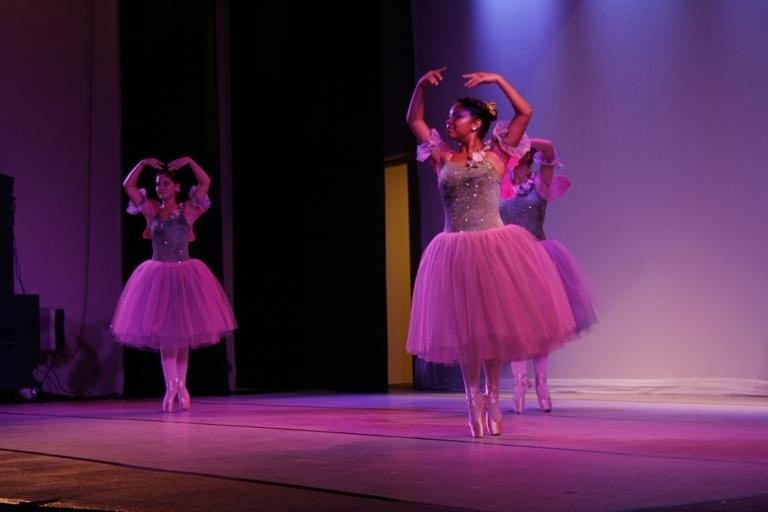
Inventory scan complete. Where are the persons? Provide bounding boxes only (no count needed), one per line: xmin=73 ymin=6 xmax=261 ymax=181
xmin=496 ymin=135 xmax=601 ymax=415
xmin=109 ymin=154 xmax=241 ymax=413
xmin=404 ymin=65 xmax=578 ymax=442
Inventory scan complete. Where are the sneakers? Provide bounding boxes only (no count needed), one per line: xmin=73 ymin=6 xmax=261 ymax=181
xmin=485 ymin=388 xmax=502 ymax=436
xmin=177 ymin=377 xmax=191 ymax=410
xmin=465 ymin=391 xmax=487 ymax=437
xmin=511 ymin=381 xmax=532 ymax=413
xmin=536 ymin=378 xmax=553 ymax=411
xmin=161 ymin=376 xmax=177 ymax=413
